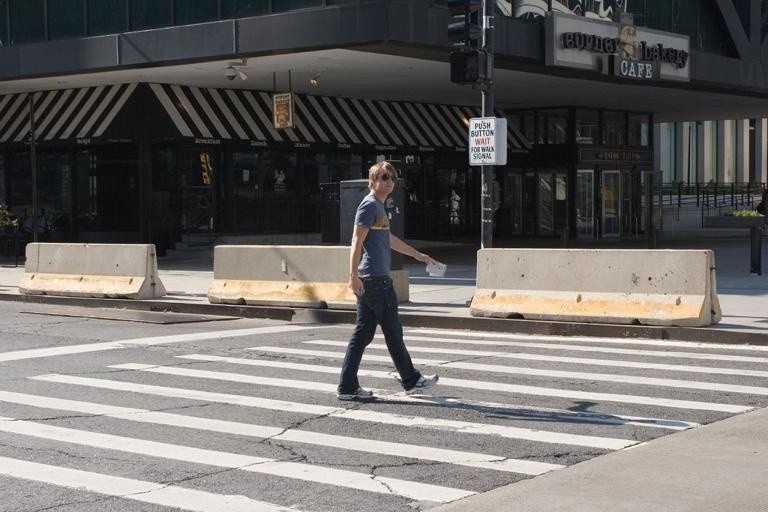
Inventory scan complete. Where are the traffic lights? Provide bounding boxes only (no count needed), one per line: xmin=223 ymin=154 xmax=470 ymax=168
xmin=446 ymin=0 xmax=483 ymax=48
xmin=448 ymin=46 xmax=494 ymax=86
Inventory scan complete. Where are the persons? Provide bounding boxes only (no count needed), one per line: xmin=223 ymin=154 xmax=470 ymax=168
xmin=335 ymin=160 xmax=439 ymax=402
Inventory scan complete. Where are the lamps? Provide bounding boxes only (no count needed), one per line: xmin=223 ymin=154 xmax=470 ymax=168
xmin=311 ymin=76 xmax=320 ymax=88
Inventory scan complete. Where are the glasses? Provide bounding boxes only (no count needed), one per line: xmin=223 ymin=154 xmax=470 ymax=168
xmin=382 ymin=173 xmax=398 ymax=183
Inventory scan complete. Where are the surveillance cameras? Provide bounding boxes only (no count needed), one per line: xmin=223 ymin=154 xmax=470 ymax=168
xmin=224 ymin=67 xmax=237 ymax=80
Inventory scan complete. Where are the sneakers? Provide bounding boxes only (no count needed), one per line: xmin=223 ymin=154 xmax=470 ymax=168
xmin=404 ymin=374 xmax=439 ymax=396
xmin=337 ymin=386 xmax=373 ymax=400
